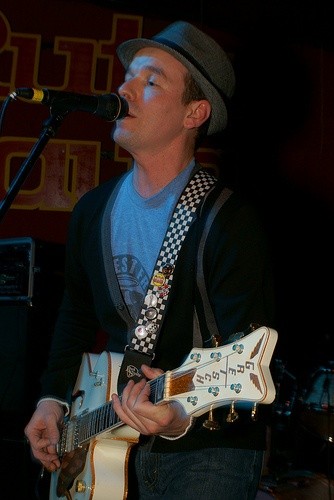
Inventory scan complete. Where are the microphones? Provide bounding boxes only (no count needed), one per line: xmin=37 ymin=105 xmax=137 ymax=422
xmin=14 ymin=88 xmax=129 ymax=123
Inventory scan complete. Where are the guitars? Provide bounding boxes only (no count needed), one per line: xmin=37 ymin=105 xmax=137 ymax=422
xmin=48 ymin=323 xmax=279 ymax=500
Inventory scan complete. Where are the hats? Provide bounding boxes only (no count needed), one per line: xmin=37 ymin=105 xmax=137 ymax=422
xmin=117 ymin=21 xmax=234 ymax=138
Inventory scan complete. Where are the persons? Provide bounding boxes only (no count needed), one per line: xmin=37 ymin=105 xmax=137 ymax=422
xmin=23 ymin=19 xmax=300 ymax=500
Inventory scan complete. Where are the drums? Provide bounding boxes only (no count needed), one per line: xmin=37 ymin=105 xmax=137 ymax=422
xmin=294 ymin=362 xmax=334 ymax=443
xmin=256 ymin=469 xmax=334 ymax=500
